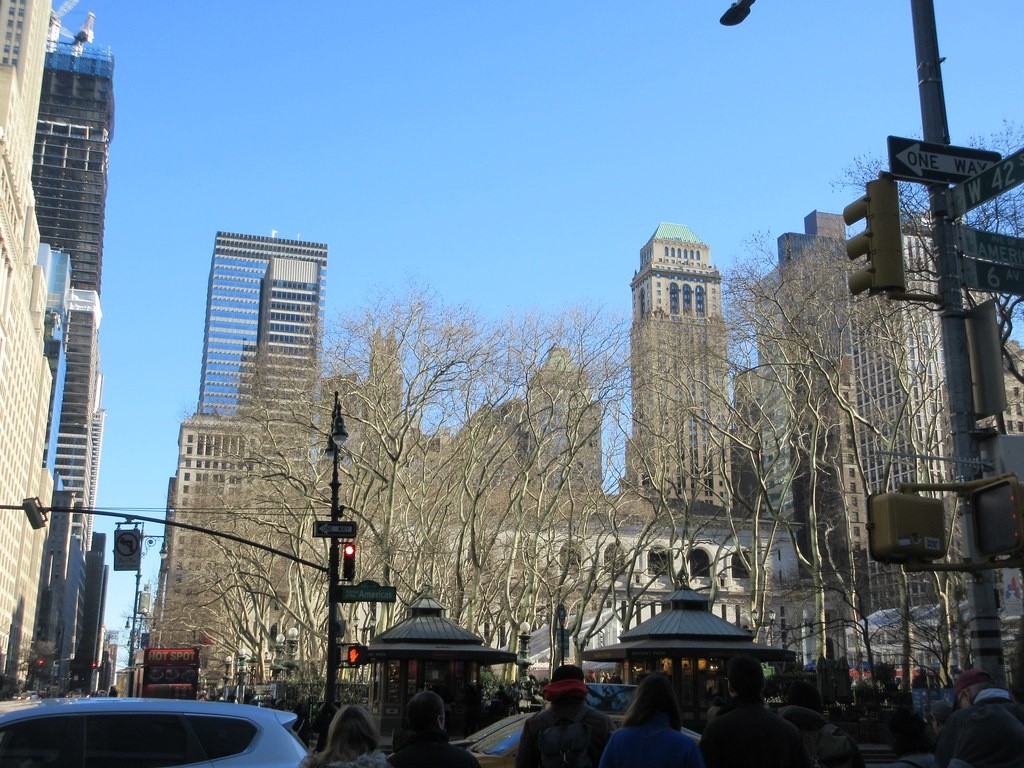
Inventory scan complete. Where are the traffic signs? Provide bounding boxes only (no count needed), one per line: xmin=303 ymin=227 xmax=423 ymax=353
xmin=887 ymin=134 xmax=1024 ymax=295
xmin=312 ymin=520 xmax=357 ymax=539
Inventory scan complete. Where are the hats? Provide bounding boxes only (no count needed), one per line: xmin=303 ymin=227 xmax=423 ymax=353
xmin=543 ymin=665 xmax=588 ymax=701
xmin=787 ymin=679 xmax=823 ymax=712
xmin=930 ymin=701 xmax=952 ymax=722
xmin=952 ymin=669 xmax=995 ymax=711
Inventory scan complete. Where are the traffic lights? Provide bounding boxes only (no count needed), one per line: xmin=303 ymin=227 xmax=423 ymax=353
xmin=969 ymin=477 xmax=1024 ymax=555
xmin=843 ymin=180 xmax=907 ymax=295
xmin=348 ymin=646 xmax=368 ymax=666
xmin=343 ymin=543 xmax=356 ymax=579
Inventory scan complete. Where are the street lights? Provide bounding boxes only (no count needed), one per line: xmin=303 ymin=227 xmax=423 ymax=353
xmin=127 ymin=534 xmax=168 ymax=698
xmin=720 ymin=1 xmax=1004 ymax=685
xmin=515 ymin=622 xmax=535 ymax=682
xmin=125 ymin=617 xmax=156 ymax=664
xmin=751 ymin=608 xmax=776 ymax=646
xmin=323 ymin=388 xmax=348 ymax=721
xmin=222 ymin=628 xmax=299 ymax=703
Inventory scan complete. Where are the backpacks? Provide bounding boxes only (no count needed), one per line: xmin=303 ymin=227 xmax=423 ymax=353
xmin=537 ymin=705 xmax=595 ymax=768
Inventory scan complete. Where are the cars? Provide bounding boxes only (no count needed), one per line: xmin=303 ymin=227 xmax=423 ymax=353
xmin=40 ymin=688 xmax=50 ymax=696
xmin=28 ymin=691 xmax=37 ymax=699
xmin=465 ymin=713 xmax=702 ymax=768
xmin=0 ymin=697 xmax=313 ymax=768
xmin=65 ymin=691 xmax=82 ymax=698
xmin=97 ymin=690 xmax=107 ymax=697
xmin=449 ymin=713 xmax=533 ymax=750
xmin=16 ymin=693 xmax=32 ymax=700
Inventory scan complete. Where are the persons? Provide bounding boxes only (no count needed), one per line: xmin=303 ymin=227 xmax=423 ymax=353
xmin=585 ymin=670 xmax=649 ymax=685
xmin=299 ymin=705 xmax=394 ymax=768
xmin=881 ymin=704 xmax=934 ymax=768
xmin=463 ymin=684 xmax=515 ymax=739
xmin=388 ymin=691 xmax=483 ymax=768
xmin=777 ymin=679 xmax=867 ymax=768
xmin=516 ymin=665 xmax=609 ymax=768
xmin=931 ymin=669 xmax=1024 ymax=768
xmin=0 ymin=686 xmax=117 ymax=701
xmin=599 ymin=673 xmax=705 ymax=768
xmin=699 ymin=656 xmax=809 ymax=768
xmin=706 ymin=696 xmax=734 ymax=725
xmin=197 ymin=692 xmax=339 ymax=754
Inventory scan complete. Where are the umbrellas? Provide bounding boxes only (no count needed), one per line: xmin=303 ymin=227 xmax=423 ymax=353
xmin=816 ymin=655 xmax=852 ymax=710
